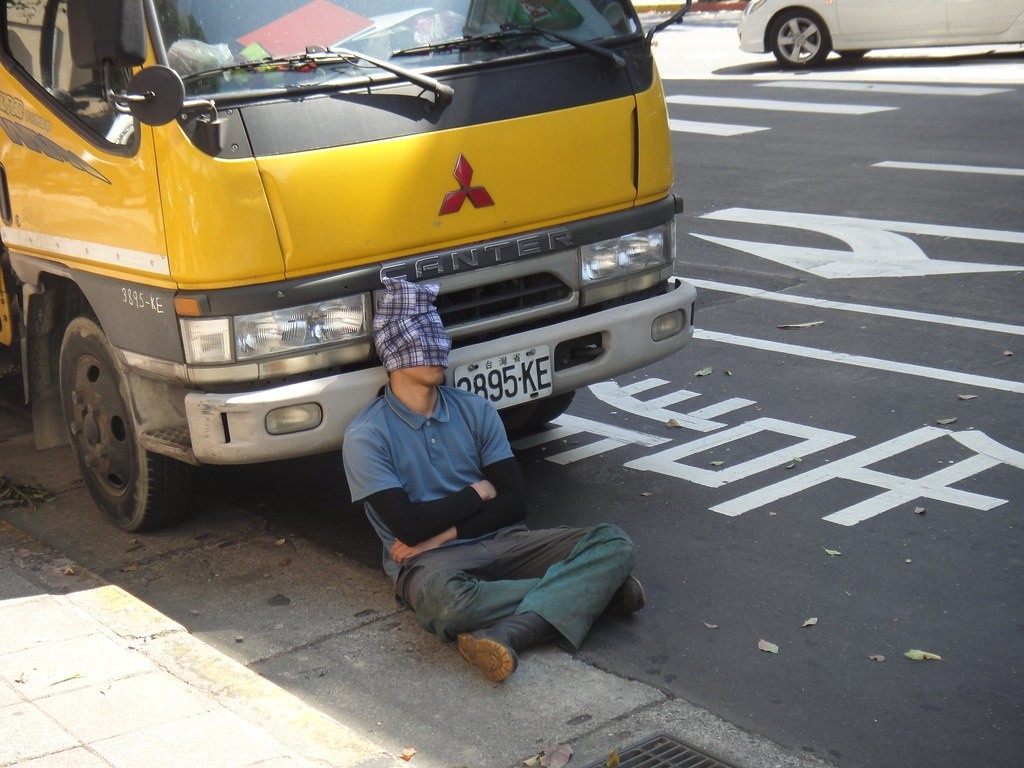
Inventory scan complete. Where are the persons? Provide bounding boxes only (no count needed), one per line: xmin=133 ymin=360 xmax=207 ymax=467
xmin=343 ymin=278 xmax=646 ymax=682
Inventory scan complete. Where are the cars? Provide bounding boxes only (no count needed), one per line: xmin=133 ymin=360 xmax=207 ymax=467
xmin=738 ymin=1 xmax=1024 ymax=68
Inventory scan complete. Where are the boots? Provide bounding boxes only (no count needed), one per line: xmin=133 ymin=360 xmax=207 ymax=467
xmin=457 ymin=610 xmax=556 ymax=684
xmin=607 ymin=576 xmax=645 ymax=617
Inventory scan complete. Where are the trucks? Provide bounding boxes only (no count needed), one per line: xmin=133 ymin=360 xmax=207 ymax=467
xmin=0 ymin=0 xmax=691 ymax=532
